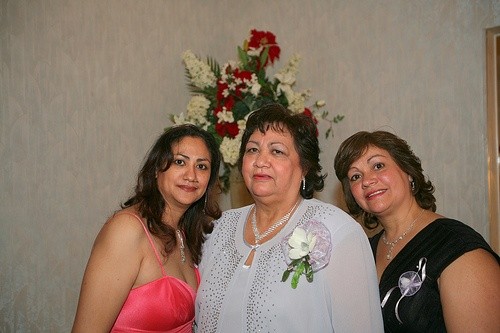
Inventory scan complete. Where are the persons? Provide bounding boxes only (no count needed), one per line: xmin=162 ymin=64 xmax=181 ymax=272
xmin=70 ymin=124 xmax=223 ymax=333
xmin=193 ymin=103 xmax=385 ymax=333
xmin=333 ymin=131 xmax=500 ymax=333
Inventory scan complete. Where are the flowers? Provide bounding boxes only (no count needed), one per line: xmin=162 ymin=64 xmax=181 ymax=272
xmin=164 ymin=29 xmax=345 ymax=195
xmin=287 ymin=227 xmax=317 ymax=260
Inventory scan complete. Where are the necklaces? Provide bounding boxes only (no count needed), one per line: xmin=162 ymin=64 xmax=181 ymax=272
xmin=174 ymin=228 xmax=186 ymax=263
xmin=250 ymin=192 xmax=301 ymax=243
xmin=382 ymin=209 xmax=425 ymax=260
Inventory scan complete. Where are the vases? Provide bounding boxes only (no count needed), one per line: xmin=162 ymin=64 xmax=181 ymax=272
xmin=224 ymin=160 xmax=255 ymax=211
